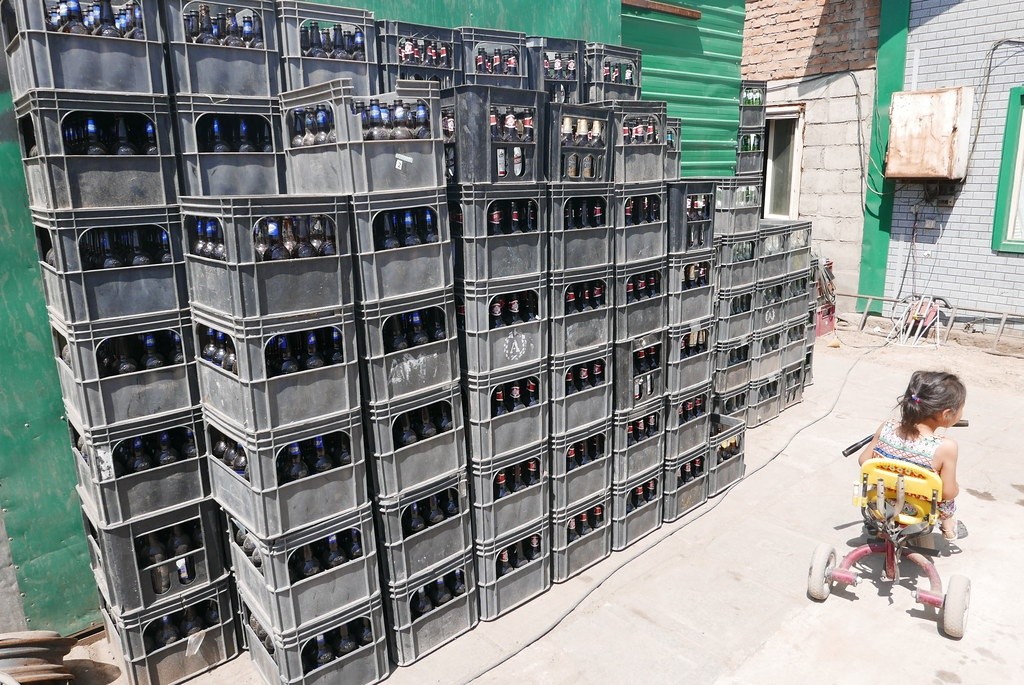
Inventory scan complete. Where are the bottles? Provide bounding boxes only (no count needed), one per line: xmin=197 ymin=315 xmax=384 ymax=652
xmin=13 ymin=0 xmax=836 ymax=670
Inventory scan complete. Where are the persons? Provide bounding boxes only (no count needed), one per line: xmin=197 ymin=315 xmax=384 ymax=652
xmin=859 ymin=371 xmax=966 ymax=542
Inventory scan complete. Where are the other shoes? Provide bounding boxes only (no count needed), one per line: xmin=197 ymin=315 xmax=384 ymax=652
xmin=866 ymin=521 xmax=878 ymax=536
xmin=942 ymin=518 xmax=957 ymax=542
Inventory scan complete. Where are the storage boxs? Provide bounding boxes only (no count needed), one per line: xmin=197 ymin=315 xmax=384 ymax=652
xmin=0 ymin=0 xmax=837 ymax=685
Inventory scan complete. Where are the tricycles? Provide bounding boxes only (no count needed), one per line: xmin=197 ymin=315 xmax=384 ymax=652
xmin=805 ymin=418 xmax=972 ymax=638
